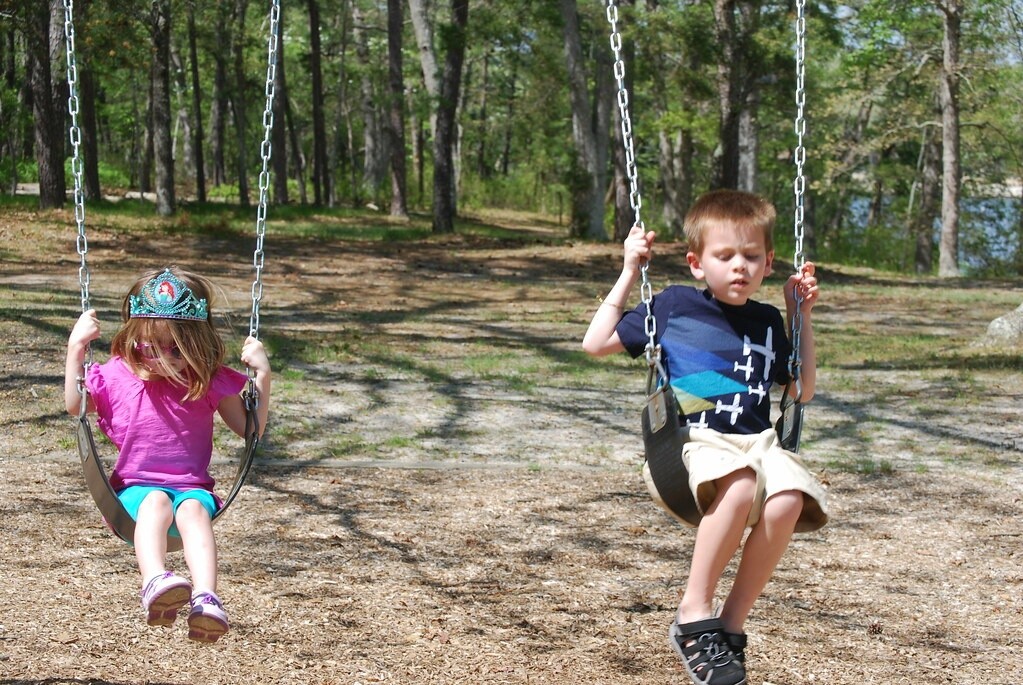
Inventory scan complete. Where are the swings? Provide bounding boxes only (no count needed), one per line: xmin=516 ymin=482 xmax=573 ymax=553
xmin=58 ymin=2 xmax=285 ymax=545
xmin=600 ymin=2 xmax=809 ymax=534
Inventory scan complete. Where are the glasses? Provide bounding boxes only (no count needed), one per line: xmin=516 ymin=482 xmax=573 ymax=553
xmin=133 ymin=339 xmax=190 ymax=361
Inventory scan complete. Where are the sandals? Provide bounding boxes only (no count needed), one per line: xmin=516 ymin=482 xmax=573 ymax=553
xmin=668 ymin=604 xmax=748 ymax=684
xmin=714 ymin=603 xmax=748 ymax=663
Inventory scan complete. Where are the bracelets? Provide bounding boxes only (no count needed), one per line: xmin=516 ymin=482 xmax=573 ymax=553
xmin=602 ymin=301 xmax=624 ymax=310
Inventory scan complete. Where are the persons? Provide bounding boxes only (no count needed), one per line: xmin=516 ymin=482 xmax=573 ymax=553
xmin=582 ymin=191 xmax=829 ymax=685
xmin=64 ymin=265 xmax=272 ymax=644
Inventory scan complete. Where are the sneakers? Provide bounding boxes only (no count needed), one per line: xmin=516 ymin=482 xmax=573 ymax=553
xmin=187 ymin=590 xmax=229 ymax=643
xmin=138 ymin=571 xmax=193 ymax=628
